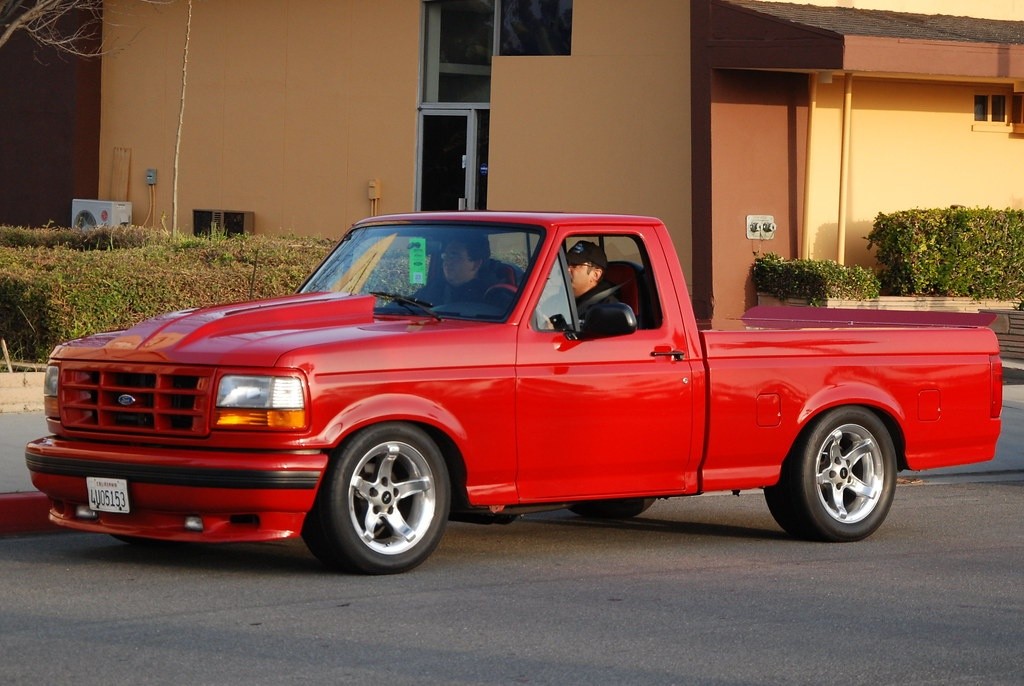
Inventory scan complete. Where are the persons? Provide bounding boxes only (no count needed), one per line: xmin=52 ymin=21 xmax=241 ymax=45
xmin=385 ymin=231 xmax=502 ymax=312
xmin=541 ymin=240 xmax=625 ymax=325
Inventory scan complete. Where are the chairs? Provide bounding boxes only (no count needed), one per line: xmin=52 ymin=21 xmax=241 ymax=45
xmin=603 ymin=261 xmax=647 ymax=330
xmin=494 ymin=261 xmax=528 ymax=310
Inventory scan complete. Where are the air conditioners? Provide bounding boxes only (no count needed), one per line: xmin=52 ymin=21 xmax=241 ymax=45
xmin=70 ymin=197 xmax=133 ymax=232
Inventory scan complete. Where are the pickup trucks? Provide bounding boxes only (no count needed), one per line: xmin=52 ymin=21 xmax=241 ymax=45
xmin=25 ymin=209 xmax=1005 ymax=576
